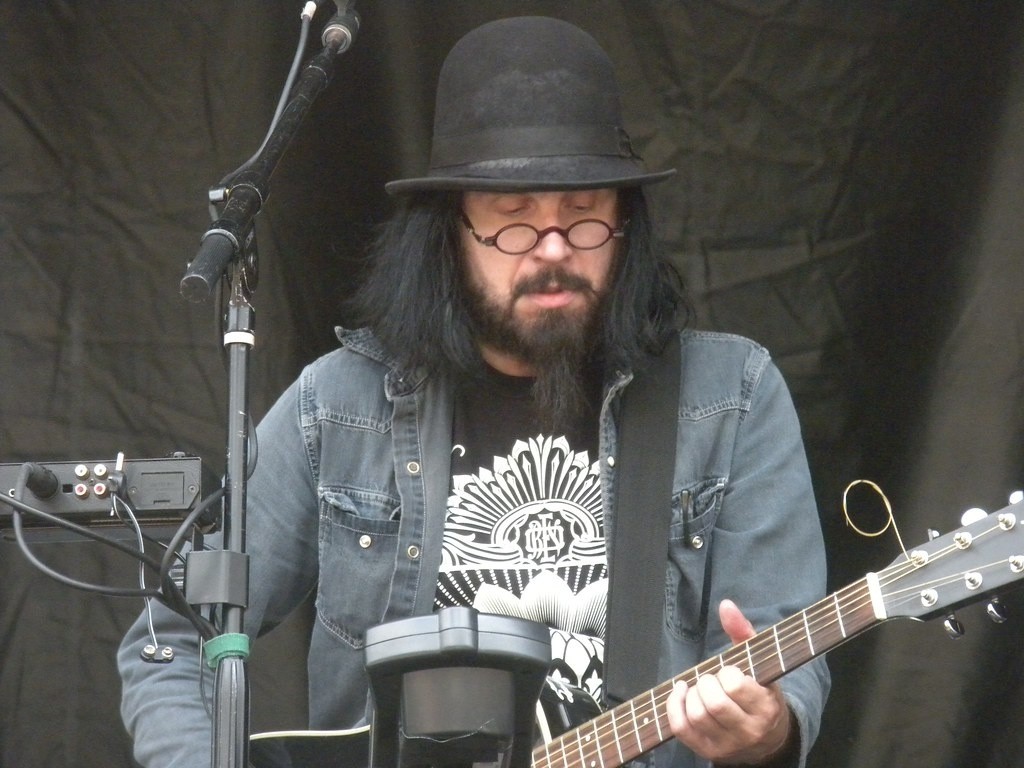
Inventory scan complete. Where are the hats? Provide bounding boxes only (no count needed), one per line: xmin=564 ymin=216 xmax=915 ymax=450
xmin=384 ymin=16 xmax=676 ymax=197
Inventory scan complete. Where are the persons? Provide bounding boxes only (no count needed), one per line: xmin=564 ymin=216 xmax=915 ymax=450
xmin=118 ymin=17 xmax=832 ymax=767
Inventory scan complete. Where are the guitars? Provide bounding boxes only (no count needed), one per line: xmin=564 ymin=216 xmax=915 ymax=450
xmin=527 ymin=486 xmax=1024 ymax=768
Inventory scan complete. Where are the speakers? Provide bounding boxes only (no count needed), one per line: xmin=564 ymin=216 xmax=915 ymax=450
xmin=0 ymin=522 xmax=204 ymax=768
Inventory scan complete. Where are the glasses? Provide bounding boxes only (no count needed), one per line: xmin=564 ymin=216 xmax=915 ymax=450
xmin=457 ymin=206 xmax=629 ymax=255
